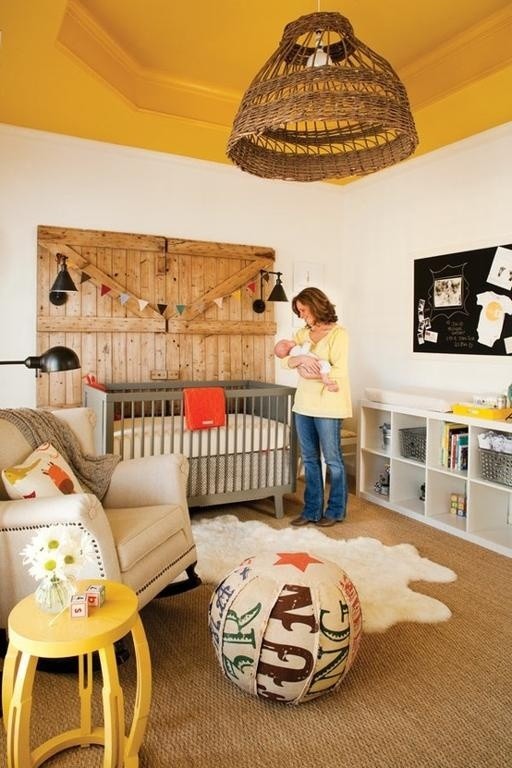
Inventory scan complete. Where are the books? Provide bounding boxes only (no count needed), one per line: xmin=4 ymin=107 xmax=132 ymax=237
xmin=440 ymin=422 xmax=467 ymax=471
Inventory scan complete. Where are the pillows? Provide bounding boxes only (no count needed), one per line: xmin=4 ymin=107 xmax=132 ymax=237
xmin=2 ymin=443 xmax=84 ymax=500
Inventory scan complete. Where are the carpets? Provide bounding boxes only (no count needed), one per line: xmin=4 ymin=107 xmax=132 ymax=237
xmin=171 ymin=514 xmax=458 ymax=633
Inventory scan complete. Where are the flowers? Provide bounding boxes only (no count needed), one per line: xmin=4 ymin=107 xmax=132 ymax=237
xmin=19 ymin=524 xmax=85 ymax=608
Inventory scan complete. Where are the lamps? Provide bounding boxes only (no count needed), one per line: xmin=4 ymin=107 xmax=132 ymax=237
xmin=0 ymin=346 xmax=81 ymax=373
xmin=253 ymin=270 xmax=288 ymax=313
xmin=226 ymin=2 xmax=418 ymax=181
xmin=49 ymin=253 xmax=78 ymax=306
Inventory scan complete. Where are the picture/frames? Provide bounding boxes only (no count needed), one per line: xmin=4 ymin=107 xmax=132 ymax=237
xmin=432 ymin=275 xmax=464 ymax=311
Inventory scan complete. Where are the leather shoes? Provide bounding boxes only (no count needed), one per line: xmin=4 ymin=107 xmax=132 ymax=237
xmin=317 ymin=517 xmax=343 ymax=527
xmin=291 ymin=517 xmax=317 ymax=526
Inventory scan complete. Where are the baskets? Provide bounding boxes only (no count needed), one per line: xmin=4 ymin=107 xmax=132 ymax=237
xmin=399 ymin=427 xmax=426 ymax=463
xmin=478 ymin=448 xmax=512 ymax=487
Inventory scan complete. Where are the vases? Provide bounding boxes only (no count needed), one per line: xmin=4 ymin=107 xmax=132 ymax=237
xmin=34 ymin=578 xmax=75 ymax=614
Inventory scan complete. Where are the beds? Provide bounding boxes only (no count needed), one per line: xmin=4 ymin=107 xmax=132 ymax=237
xmin=82 ymin=380 xmax=297 ymax=519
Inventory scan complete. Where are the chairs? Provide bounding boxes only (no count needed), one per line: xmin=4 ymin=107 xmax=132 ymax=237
xmin=0 ymin=408 xmax=201 ymax=673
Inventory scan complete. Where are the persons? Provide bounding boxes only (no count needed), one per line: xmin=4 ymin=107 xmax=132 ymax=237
xmin=290 ymin=288 xmax=353 ymax=527
xmin=274 ymin=339 xmax=339 ymax=392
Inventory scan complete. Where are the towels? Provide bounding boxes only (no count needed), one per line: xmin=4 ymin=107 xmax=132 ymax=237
xmin=182 ymin=387 xmax=226 ymax=431
xmin=0 ymin=405 xmax=122 ymax=501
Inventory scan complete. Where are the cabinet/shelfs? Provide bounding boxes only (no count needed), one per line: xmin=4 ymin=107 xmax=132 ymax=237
xmin=356 ymin=400 xmax=512 ymax=559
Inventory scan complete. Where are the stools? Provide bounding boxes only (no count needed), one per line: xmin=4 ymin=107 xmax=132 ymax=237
xmin=298 ymin=428 xmax=357 ymax=490
xmin=2 ymin=579 xmax=153 ymax=767
xmin=208 ymin=550 xmax=362 ymax=705
